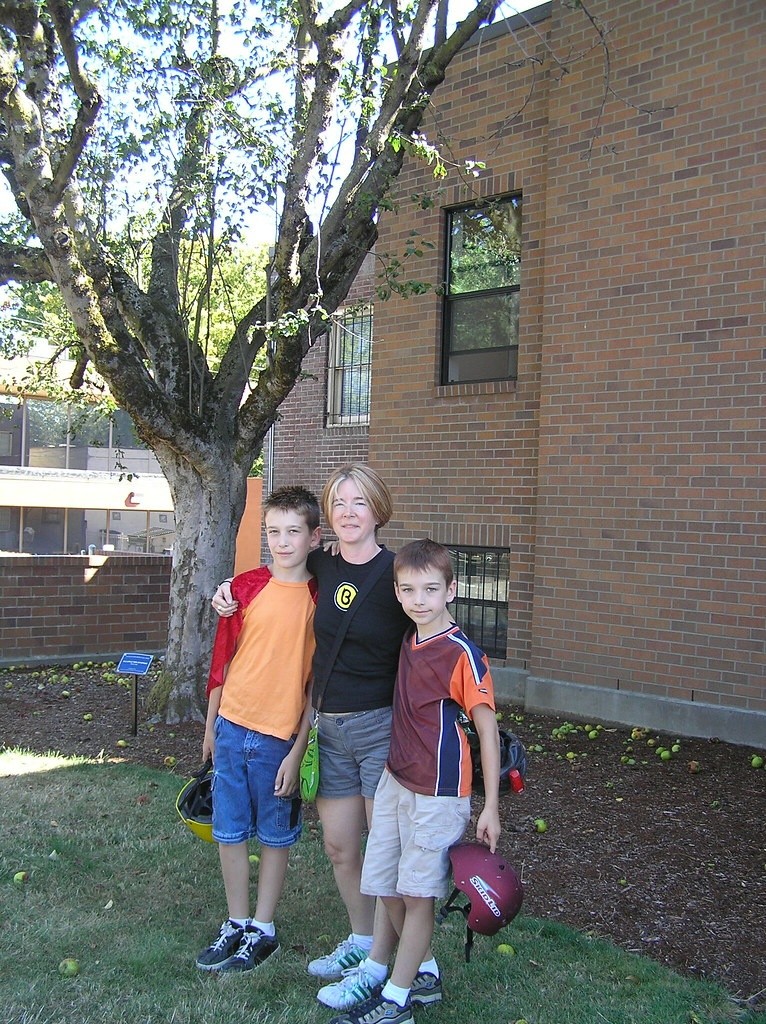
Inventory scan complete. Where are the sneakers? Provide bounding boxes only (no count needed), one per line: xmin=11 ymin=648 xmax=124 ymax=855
xmin=216 ymin=925 xmax=281 ymax=977
xmin=196 ymin=920 xmax=246 ymax=971
xmin=330 ymin=984 xmax=416 ymax=1024
xmin=308 ymin=934 xmax=370 ymax=979
xmin=408 ymin=966 xmax=442 ymax=1006
xmin=317 ymin=960 xmax=389 ymax=1010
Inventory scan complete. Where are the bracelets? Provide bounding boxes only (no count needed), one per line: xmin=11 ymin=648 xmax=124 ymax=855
xmin=217 ymin=581 xmax=232 ymax=590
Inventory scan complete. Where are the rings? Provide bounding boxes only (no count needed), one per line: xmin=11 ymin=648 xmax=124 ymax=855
xmin=218 ymin=606 xmax=222 ymax=611
xmin=218 ymin=611 xmax=221 ymax=615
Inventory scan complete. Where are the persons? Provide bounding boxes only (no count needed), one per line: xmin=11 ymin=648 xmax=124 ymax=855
xmin=196 ymin=487 xmax=321 ymax=977
xmin=212 ymin=459 xmax=418 ymax=1024
xmin=324 ymin=540 xmax=501 ymax=1024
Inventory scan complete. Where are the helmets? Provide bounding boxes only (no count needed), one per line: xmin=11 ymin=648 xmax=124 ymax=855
xmin=467 ymin=728 xmax=527 ymax=797
xmin=175 ymin=770 xmax=217 ymax=843
xmin=448 ymin=842 xmax=524 ymax=936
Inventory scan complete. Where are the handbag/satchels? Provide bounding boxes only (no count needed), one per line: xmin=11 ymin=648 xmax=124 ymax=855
xmin=299 ymin=716 xmax=320 ymax=804
